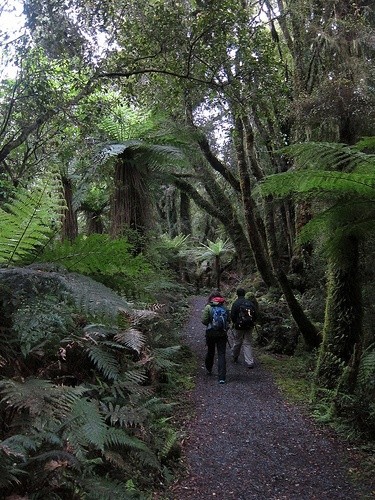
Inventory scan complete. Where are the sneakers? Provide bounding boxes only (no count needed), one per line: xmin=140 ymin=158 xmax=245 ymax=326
xmin=247 ymin=364 xmax=252 ymax=367
xmin=219 ymin=379 xmax=224 ymax=382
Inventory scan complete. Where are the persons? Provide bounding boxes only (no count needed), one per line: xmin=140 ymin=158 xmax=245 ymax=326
xmin=202 ymin=292 xmax=230 ymax=382
xmin=231 ymin=288 xmax=257 ymax=370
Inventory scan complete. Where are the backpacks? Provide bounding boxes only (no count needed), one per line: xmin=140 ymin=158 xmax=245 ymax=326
xmin=234 ymin=299 xmax=258 ymax=330
xmin=205 ymin=302 xmax=229 ymax=334
